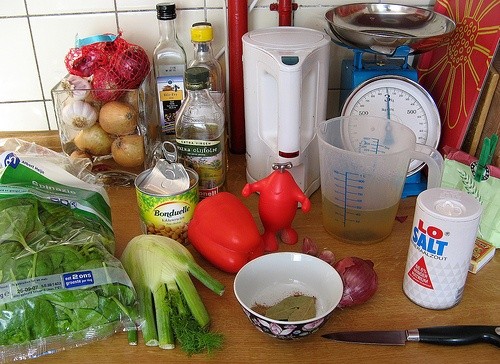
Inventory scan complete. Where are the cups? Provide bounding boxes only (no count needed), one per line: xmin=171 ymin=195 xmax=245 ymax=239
xmin=402 ymin=187 xmax=483 ymax=311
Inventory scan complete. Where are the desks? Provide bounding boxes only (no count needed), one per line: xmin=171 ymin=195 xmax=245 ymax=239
xmin=0 ymin=130 xmax=500 ymax=364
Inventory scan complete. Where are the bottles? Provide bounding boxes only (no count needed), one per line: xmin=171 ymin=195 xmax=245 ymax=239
xmin=174 ymin=66 xmax=228 ymax=202
xmin=153 ymin=3 xmax=188 ymax=126
xmin=186 ymin=22 xmax=222 ymax=95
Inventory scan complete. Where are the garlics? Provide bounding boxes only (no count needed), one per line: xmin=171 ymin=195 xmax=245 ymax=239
xmin=62 ymin=101 xmax=97 ymax=130
xmin=61 ymin=75 xmax=92 ymax=100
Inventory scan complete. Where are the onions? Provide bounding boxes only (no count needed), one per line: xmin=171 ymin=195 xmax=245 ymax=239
xmin=303 ymin=237 xmax=377 ymax=308
xmin=61 ymin=54 xmax=157 ymax=168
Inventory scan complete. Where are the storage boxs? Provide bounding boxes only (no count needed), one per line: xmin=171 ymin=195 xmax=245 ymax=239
xmin=469 ymin=238 xmax=496 ymax=274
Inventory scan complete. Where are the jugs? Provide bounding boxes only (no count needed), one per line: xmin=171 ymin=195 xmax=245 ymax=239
xmin=316 ymin=115 xmax=445 ymax=245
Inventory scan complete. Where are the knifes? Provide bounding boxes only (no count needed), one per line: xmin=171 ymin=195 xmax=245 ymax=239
xmin=320 ymin=325 xmax=500 ymax=348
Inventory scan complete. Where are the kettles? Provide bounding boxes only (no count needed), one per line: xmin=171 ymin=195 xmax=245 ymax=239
xmin=242 ymin=27 xmax=330 ymax=208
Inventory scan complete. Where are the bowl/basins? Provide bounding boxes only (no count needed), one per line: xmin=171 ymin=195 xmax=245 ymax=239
xmin=233 ymin=251 xmax=344 ymax=342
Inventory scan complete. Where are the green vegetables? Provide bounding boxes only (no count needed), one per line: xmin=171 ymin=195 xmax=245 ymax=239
xmin=0 ymin=196 xmax=137 ymax=347
xmin=163 ymin=291 xmax=225 ymax=359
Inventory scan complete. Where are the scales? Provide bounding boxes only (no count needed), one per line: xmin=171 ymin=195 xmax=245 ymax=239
xmin=322 ymin=2 xmax=456 ymax=198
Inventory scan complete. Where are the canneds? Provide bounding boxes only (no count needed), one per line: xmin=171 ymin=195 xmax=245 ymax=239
xmin=402 ymin=188 xmax=483 ymax=310
xmin=135 ymin=141 xmax=199 ymax=245
